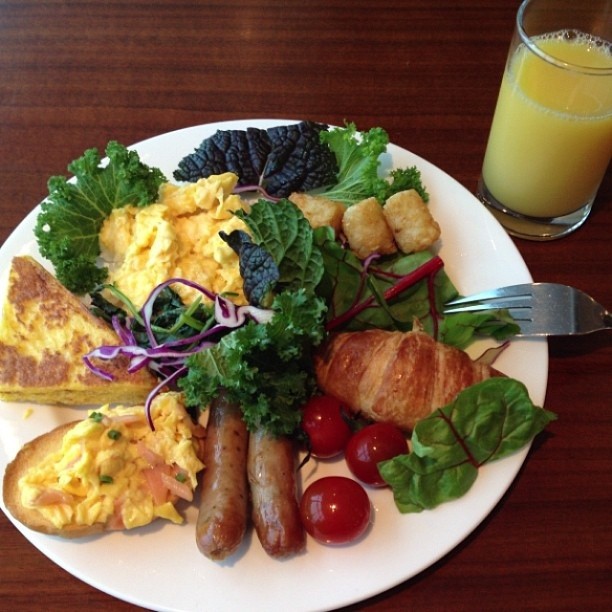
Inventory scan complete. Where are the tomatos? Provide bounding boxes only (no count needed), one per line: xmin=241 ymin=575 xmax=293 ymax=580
xmin=345 ymin=421 xmax=408 ymax=489
xmin=294 ymin=394 xmax=353 ymax=460
xmin=298 ymin=475 xmax=371 ymax=547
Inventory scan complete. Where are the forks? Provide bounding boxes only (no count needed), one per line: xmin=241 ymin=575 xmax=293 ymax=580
xmin=442 ymin=282 xmax=612 ymax=340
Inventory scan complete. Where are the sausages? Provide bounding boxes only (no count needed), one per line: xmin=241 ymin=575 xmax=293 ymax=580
xmin=247 ymin=420 xmax=304 ymax=557
xmin=196 ymin=403 xmax=247 ymax=557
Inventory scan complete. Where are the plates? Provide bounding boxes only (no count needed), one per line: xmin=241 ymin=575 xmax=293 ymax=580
xmin=0 ymin=119 xmax=550 ymax=612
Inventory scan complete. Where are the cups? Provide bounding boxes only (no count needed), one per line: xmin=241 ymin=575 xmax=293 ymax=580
xmin=477 ymin=1 xmax=612 ymax=242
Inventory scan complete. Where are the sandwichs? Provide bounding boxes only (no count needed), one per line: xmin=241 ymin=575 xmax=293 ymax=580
xmin=1 ymin=253 xmax=163 ymax=405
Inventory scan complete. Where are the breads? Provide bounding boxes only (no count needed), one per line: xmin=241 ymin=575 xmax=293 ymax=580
xmin=312 ymin=330 xmax=512 ymax=435
xmin=3 ymin=419 xmax=206 ymax=538
